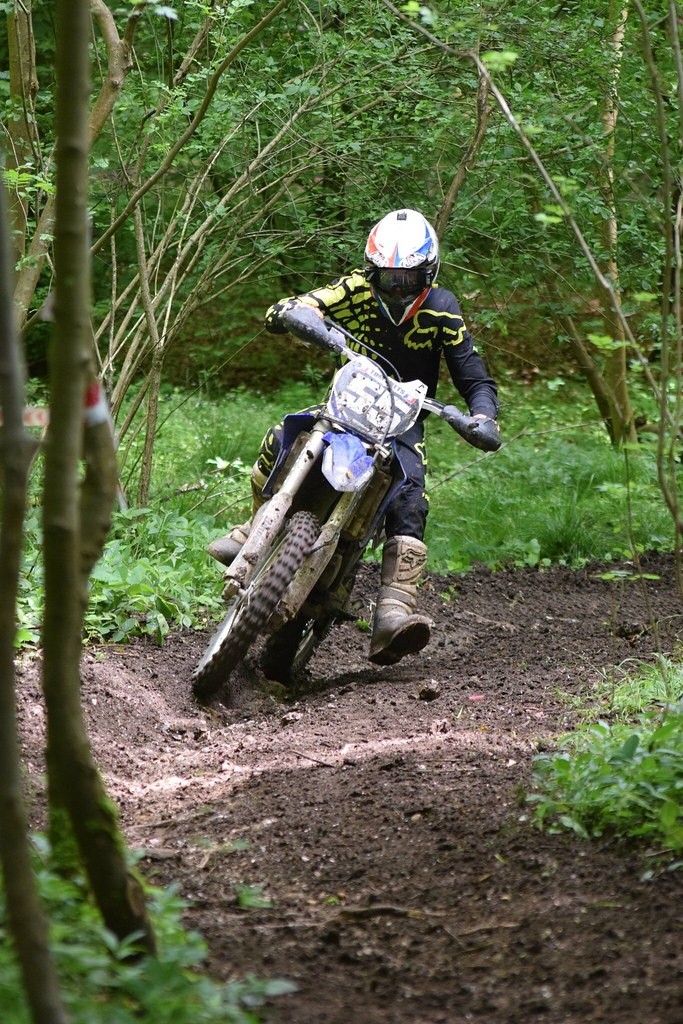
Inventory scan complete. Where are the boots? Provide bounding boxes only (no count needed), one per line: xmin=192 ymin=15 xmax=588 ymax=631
xmin=368 ymin=535 xmax=430 ymax=666
xmin=205 ymin=462 xmax=271 ymax=568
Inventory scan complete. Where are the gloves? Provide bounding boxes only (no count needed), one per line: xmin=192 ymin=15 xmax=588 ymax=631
xmin=283 ymin=303 xmax=325 ymax=319
xmin=475 ymin=415 xmax=501 ymax=435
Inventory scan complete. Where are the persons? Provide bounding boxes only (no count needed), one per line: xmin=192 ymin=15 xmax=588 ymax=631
xmin=207 ymin=209 xmax=497 ymax=665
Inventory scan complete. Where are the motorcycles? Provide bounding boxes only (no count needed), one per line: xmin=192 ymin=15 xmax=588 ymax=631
xmin=190 ymin=308 xmax=503 ymax=707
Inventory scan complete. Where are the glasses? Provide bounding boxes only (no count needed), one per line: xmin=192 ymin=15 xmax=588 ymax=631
xmin=365 ymin=266 xmax=430 ymax=297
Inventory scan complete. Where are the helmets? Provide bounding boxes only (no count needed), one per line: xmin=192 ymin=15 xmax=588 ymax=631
xmin=363 ymin=209 xmax=439 ymax=326
